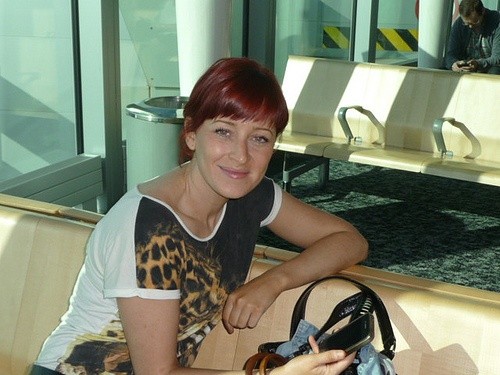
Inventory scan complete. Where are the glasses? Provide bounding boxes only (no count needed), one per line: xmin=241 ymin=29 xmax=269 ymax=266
xmin=462 ymin=14 xmax=483 ymax=26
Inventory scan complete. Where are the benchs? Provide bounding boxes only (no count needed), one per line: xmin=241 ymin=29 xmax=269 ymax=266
xmin=0 ymin=55 xmax=500 ymax=375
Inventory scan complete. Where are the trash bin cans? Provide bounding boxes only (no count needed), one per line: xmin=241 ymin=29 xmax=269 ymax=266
xmin=124 ymin=95 xmax=188 ymax=191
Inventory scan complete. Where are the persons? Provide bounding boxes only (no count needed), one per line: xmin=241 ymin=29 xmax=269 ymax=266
xmin=445 ymin=0 xmax=500 ymax=75
xmin=30 ymin=57 xmax=367 ymax=375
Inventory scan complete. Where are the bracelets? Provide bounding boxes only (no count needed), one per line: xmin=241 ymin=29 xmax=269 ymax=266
xmin=245 ymin=352 xmax=286 ymax=375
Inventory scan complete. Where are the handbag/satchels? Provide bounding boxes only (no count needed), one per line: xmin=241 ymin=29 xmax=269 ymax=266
xmin=243 ymin=276 xmax=398 ymax=375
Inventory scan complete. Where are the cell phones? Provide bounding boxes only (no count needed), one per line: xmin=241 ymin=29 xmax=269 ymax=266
xmin=460 ymin=63 xmax=468 ymax=67
xmin=319 ymin=313 xmax=374 ymax=356
